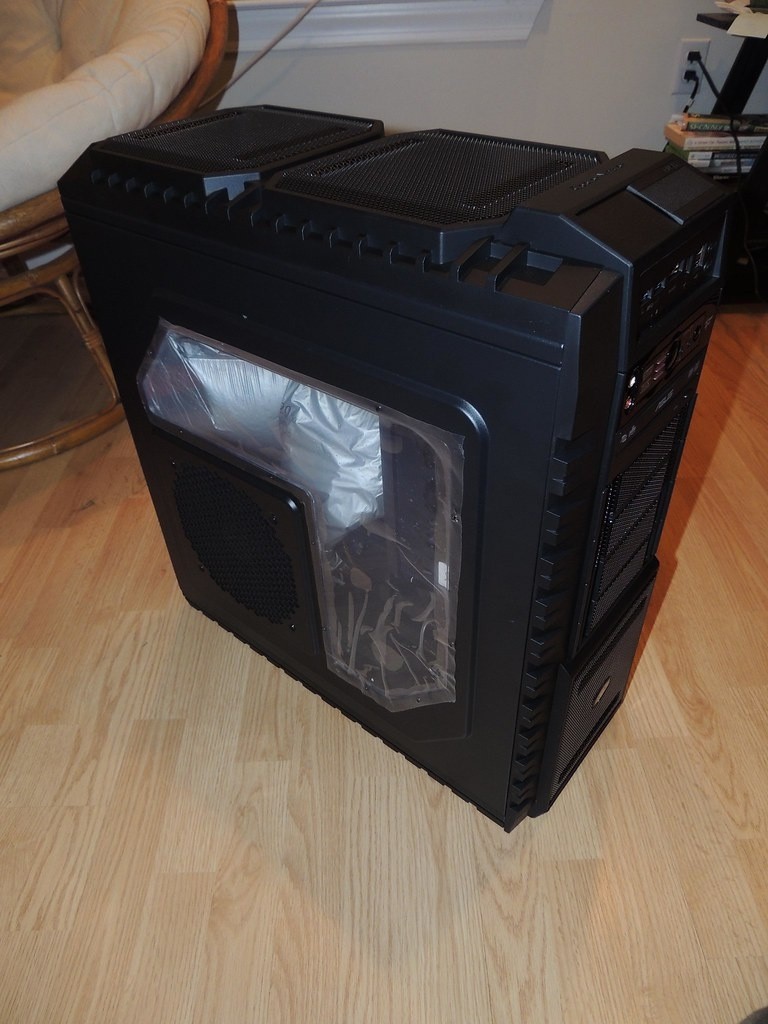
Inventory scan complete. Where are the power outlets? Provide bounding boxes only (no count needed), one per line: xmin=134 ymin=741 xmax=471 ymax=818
xmin=671 ymin=39 xmax=710 ymax=98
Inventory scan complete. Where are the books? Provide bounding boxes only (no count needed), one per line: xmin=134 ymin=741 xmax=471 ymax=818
xmin=662 ymin=111 xmax=768 ymax=180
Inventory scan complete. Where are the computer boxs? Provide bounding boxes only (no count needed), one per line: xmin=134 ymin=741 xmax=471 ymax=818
xmin=56 ymin=103 xmax=728 ymax=833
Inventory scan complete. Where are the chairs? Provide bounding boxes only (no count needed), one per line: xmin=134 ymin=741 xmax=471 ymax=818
xmin=0 ymin=0 xmax=243 ymax=479
